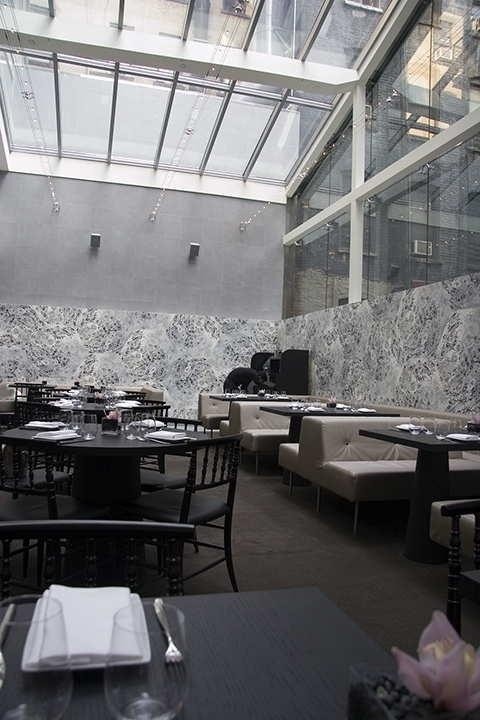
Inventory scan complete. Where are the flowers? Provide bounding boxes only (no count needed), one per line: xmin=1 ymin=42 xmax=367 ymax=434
xmin=392 ymin=609 xmax=480 ymax=716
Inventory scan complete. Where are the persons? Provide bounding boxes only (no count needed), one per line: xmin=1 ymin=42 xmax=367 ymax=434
xmin=224 ymin=367 xmax=271 ymax=395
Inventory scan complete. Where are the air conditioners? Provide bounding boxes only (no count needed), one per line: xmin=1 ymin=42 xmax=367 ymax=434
xmin=411 ymin=239 xmax=433 ymax=257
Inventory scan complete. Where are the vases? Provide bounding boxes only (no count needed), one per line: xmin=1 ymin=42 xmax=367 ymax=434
xmin=345 ymin=654 xmax=478 ymax=720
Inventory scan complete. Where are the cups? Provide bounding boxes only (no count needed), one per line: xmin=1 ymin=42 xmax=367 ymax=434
xmin=393 ymin=416 xmax=479 ymax=443
xmin=288 ymin=392 xmax=377 ymax=412
xmin=0 ymin=594 xmax=74 ymax=720
xmin=54 ymin=385 xmax=142 ymax=409
xmin=23 ymin=411 xmax=186 ymax=442
xmin=223 ymin=386 xmax=287 ymax=399
xmin=104 ymin=603 xmax=189 ymax=720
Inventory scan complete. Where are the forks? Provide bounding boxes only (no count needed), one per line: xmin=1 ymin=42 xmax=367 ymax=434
xmin=153 ymin=598 xmax=183 ymax=664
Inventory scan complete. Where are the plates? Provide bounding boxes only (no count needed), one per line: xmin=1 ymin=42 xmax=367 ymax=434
xmin=20 ymin=593 xmax=151 ymax=672
